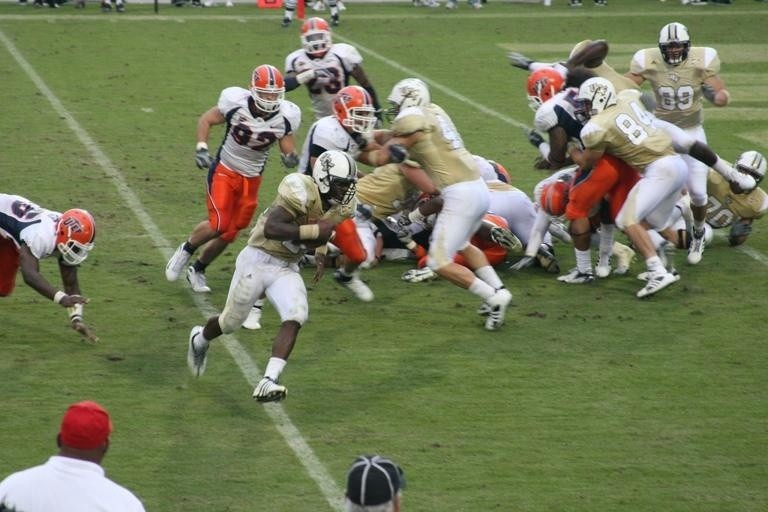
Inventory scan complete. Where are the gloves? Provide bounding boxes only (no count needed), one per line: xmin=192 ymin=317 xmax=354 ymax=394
xmin=194 ymin=148 xmax=216 ymax=170
xmin=280 ymin=151 xmax=299 ymax=168
xmin=701 ymin=83 xmax=715 ymax=102
xmin=314 ymin=67 xmax=336 ymax=80
xmin=388 ymin=144 xmax=407 ymax=163
xmin=641 ymin=92 xmax=656 ymax=112
xmin=396 ymin=209 xmax=414 ymax=244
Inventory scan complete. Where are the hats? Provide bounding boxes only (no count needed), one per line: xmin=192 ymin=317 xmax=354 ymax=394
xmin=59 ymin=400 xmax=112 ymax=449
xmin=345 ymin=455 xmax=404 ymax=505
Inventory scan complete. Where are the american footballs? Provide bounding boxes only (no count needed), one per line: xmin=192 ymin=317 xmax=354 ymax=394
xmin=291 ymin=216 xmax=323 ymax=247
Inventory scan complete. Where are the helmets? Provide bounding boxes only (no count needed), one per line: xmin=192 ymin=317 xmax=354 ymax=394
xmin=55 ymin=208 xmax=96 ymax=266
xmin=249 ymin=64 xmax=285 ymax=113
xmin=331 ymin=78 xmax=431 ymax=134
xmin=299 ymin=17 xmax=332 ymax=54
xmin=526 ymin=67 xmax=617 ymax=125
xmin=539 ymin=179 xmax=570 ymax=218
xmin=312 ymin=150 xmax=358 ymax=206
xmin=658 ymin=22 xmax=691 ymax=66
xmin=734 ymin=150 xmax=768 ymax=183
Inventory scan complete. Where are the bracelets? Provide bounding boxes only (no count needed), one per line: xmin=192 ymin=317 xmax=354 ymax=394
xmin=53 ymin=291 xmax=67 ymax=304
xmin=66 ymin=303 xmax=85 ymax=321
xmin=196 ymin=142 xmax=209 ymax=151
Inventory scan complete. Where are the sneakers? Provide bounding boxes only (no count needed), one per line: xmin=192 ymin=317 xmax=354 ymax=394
xmin=489 ymin=227 xmax=523 ymax=253
xmin=334 ymin=267 xmax=375 ymax=302
xmin=186 ymin=325 xmax=210 ymax=377
xmin=477 ymin=286 xmax=513 ymax=330
xmin=401 ymin=266 xmax=439 ymax=282
xmin=636 ymin=226 xmax=706 ymax=298
xmin=165 ymin=241 xmax=212 ymax=292
xmin=242 ymin=299 xmax=265 ymax=330
xmin=252 ymin=378 xmax=288 ymax=402
xmin=722 ymin=167 xmax=757 ymax=190
xmin=538 ymin=241 xmax=636 ymax=284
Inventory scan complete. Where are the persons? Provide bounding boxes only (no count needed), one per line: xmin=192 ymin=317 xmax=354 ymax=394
xmin=187 ymin=150 xmax=360 ymax=403
xmin=342 ymin=453 xmax=406 ymax=512
xmin=162 ymin=62 xmax=301 ymax=293
xmin=1 ymin=189 xmax=102 ymax=344
xmin=281 ymin=16 xmax=560 ymax=333
xmin=281 ymin=0 xmax=345 ymax=26
xmin=0 ymin=400 xmax=147 ymax=512
xmin=411 ymin=1 xmax=483 ymax=9
xmin=508 ymin=22 xmax=768 ymax=300
